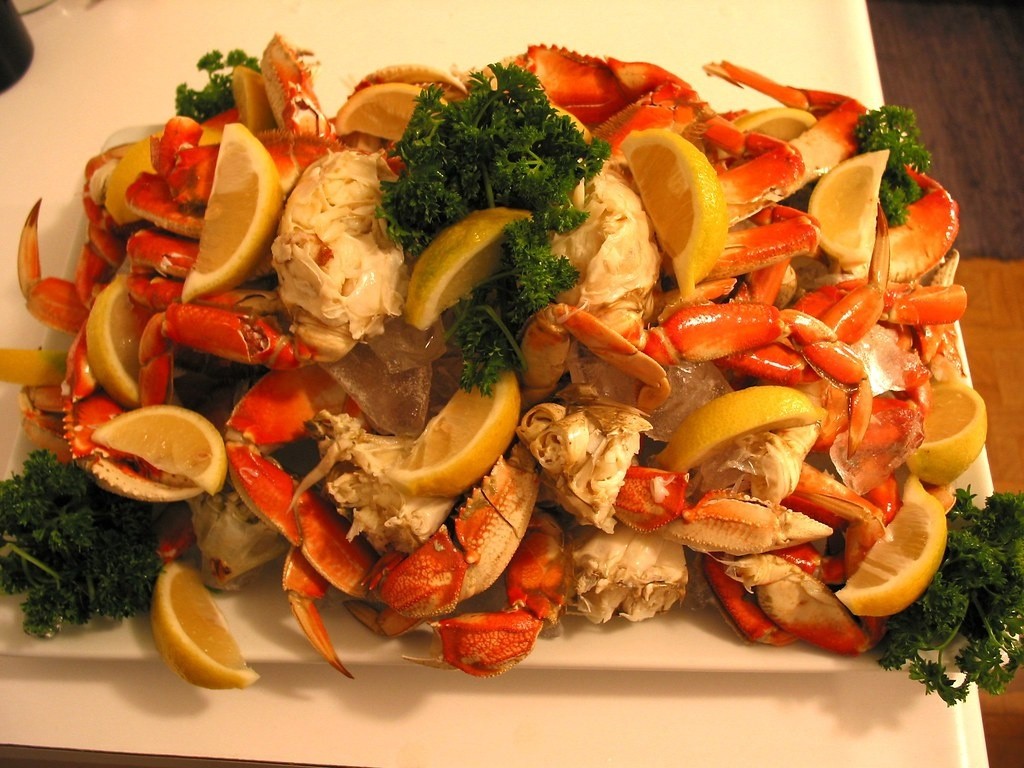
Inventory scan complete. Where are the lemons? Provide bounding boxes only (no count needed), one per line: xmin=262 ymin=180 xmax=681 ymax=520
xmin=620 ymin=127 xmax=728 ymax=297
xmin=150 ymin=558 xmax=259 ymax=691
xmin=230 ymin=65 xmax=278 ymax=130
xmin=807 ymin=149 xmax=890 ymax=263
xmin=335 ymin=82 xmax=447 ymax=140
xmin=180 ymin=122 xmax=283 ymax=304
xmin=906 ymin=381 xmax=988 ymax=486
xmin=91 ymin=404 xmax=227 ymax=497
xmin=717 ymin=107 xmax=817 ymax=160
xmin=834 ymin=473 xmax=947 ymax=616
xmin=656 ymin=384 xmax=819 ymax=471
xmin=389 ymin=367 xmax=520 ymax=498
xmin=0 ymin=347 xmax=69 ymax=386
xmin=406 ymin=206 xmax=533 ymax=331
xmin=86 ymin=276 xmax=142 ymax=410
xmin=105 ymin=119 xmax=228 ymax=225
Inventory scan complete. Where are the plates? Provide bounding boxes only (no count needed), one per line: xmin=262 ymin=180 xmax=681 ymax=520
xmin=0 ymin=0 xmax=990 ymax=768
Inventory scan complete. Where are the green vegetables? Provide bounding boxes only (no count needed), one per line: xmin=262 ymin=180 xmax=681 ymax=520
xmin=854 ymin=104 xmax=933 ymax=226
xmin=872 ymin=482 xmax=1024 ymax=708
xmin=374 ymin=61 xmax=611 ymax=399
xmin=174 ymin=48 xmax=266 ymax=123
xmin=0 ymin=447 xmax=165 ymax=640
